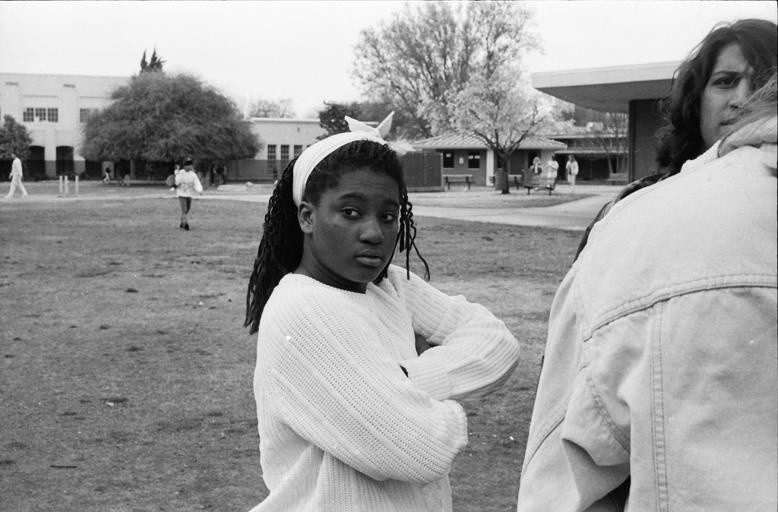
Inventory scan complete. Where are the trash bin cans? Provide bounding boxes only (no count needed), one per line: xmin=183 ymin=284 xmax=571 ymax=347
xmin=495 ymin=167 xmax=508 ymax=191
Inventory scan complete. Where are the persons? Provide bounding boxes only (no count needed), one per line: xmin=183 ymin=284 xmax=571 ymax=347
xmin=103 ymin=163 xmax=112 ymax=182
xmin=545 ymin=152 xmax=559 ymax=193
xmin=566 ymin=154 xmax=579 ymax=192
xmin=517 ymin=71 xmax=778 ymax=512
xmin=243 ymin=109 xmax=520 ymax=511
xmin=573 ymin=15 xmax=778 ymax=265
xmin=168 ymin=157 xmax=204 ymax=229
xmin=4 ymin=151 xmax=30 ymax=198
xmin=529 ymin=156 xmax=543 ymax=175
xmin=210 ymin=162 xmax=229 ymax=187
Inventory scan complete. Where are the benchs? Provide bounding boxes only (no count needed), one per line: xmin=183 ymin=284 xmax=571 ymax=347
xmin=489 ymin=175 xmax=522 ymax=190
xmin=442 ymin=174 xmax=475 ymax=191
xmin=607 ymin=174 xmax=627 ymax=185
xmin=524 ymin=174 xmax=558 ymax=195
